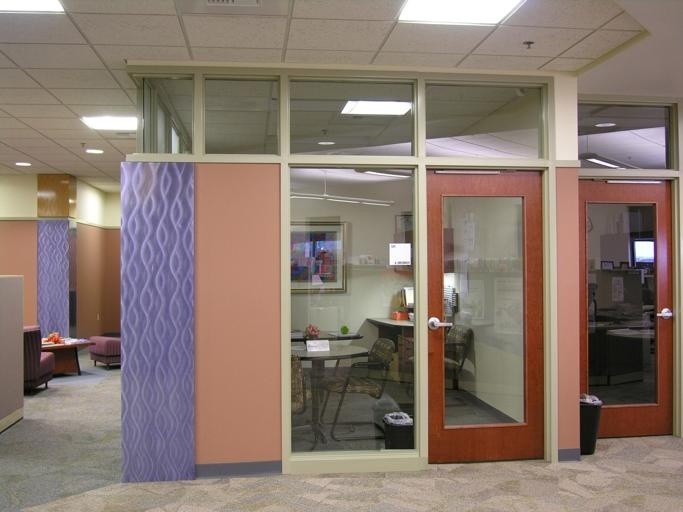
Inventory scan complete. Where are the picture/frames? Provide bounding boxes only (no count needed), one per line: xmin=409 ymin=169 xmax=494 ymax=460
xmin=291 ymin=221 xmax=349 ymax=293
xmin=392 ymin=213 xmax=414 ymax=272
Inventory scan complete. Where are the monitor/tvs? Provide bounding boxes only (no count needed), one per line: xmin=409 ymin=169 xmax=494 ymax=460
xmin=632 ymin=238 xmax=656 ymax=267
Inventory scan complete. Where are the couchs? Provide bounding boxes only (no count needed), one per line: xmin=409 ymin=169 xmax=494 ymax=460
xmin=23 ymin=326 xmax=55 ymax=398
xmin=89 ymin=336 xmax=121 ymax=370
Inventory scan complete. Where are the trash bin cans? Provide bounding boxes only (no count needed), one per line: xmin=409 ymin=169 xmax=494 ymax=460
xmin=382 ymin=412 xmax=414 ymax=448
xmin=580 ymin=397 xmax=602 ymax=455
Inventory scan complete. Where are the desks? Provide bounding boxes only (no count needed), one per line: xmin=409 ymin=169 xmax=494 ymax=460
xmin=287 ymin=330 xmax=369 ymax=447
xmin=367 ymin=318 xmax=455 ymax=394
xmin=588 ymin=321 xmax=656 ymax=386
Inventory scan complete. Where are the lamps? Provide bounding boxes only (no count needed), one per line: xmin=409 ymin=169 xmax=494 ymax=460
xmin=583 ymin=136 xmax=642 ymax=168
xmin=355 ymin=168 xmax=412 ymax=180
xmin=290 ymin=169 xmax=395 ymax=207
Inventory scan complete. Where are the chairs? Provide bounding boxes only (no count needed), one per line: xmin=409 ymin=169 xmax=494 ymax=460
xmin=319 ymin=337 xmax=396 ymax=442
xmin=401 ymin=324 xmax=473 ymax=399
